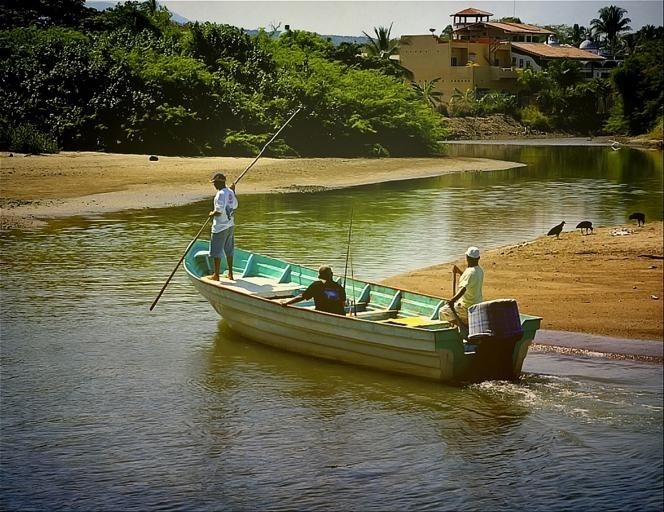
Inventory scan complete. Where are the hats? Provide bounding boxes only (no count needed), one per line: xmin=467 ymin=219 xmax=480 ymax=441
xmin=465 ymin=246 xmax=480 ymax=259
xmin=317 ymin=266 xmax=333 ymax=279
xmin=209 ymin=172 xmax=226 ymax=183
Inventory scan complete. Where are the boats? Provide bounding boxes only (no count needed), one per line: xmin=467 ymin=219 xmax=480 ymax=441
xmin=183 ymin=238 xmax=544 ymax=385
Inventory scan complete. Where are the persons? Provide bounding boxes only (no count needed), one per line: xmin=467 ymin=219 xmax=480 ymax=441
xmin=204 ymin=173 xmax=238 ymax=282
xmin=280 ymin=266 xmax=347 ymax=317
xmin=438 ymin=247 xmax=484 ymax=329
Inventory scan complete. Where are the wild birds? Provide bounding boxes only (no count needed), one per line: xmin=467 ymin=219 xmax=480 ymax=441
xmin=576 ymin=221 xmax=593 ymax=235
xmin=628 ymin=212 xmax=646 ymax=227
xmin=547 ymin=221 xmax=567 ymax=239
